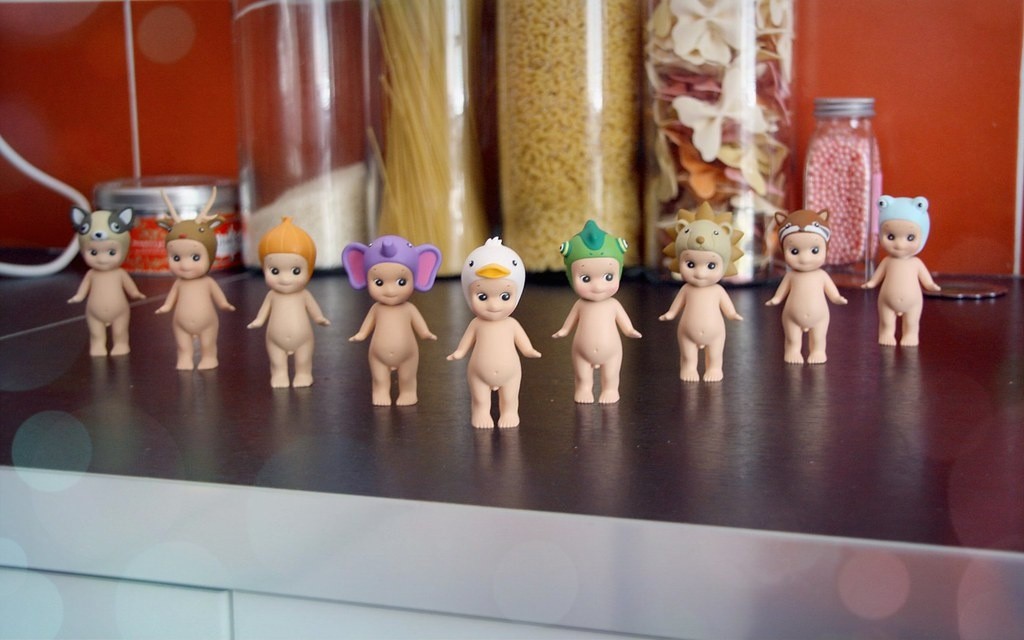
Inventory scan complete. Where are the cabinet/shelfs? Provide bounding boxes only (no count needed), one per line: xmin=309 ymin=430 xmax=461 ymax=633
xmin=0 ymin=249 xmax=1023 ymax=640
